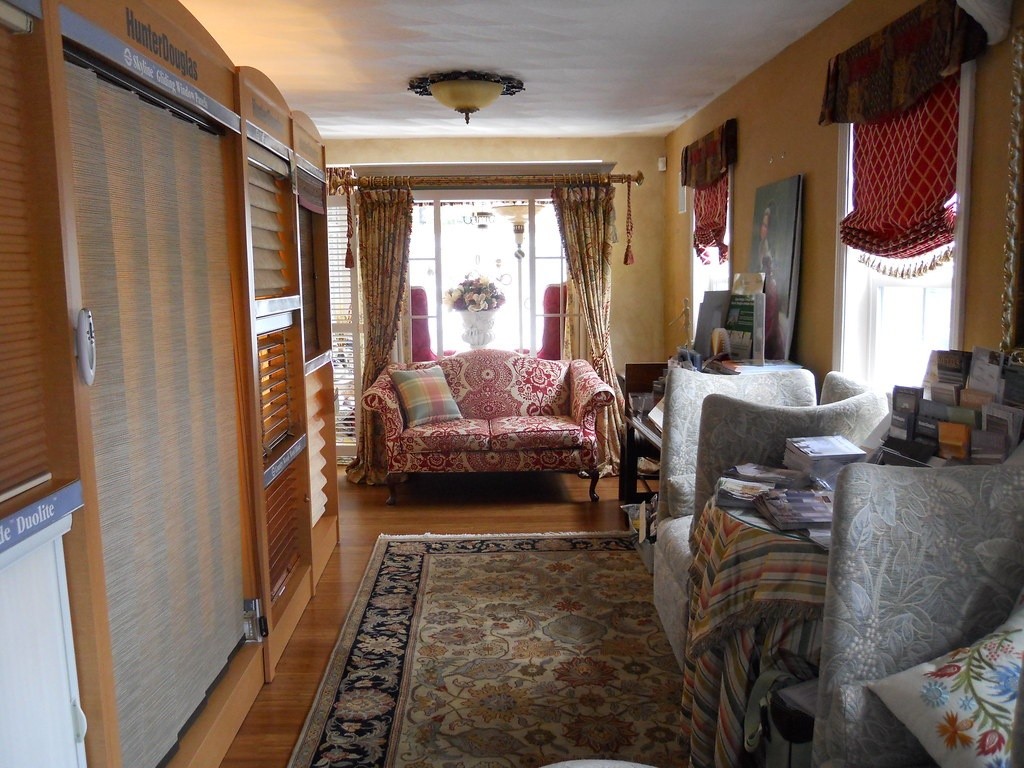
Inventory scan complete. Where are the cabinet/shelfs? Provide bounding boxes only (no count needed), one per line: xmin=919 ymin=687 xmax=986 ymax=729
xmin=1 ymin=0 xmax=340 ymax=767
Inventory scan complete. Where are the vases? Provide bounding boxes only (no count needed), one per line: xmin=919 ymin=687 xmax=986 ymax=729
xmin=457 ymin=309 xmax=500 ymax=351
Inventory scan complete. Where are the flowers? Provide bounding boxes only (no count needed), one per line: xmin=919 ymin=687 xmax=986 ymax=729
xmin=443 ymin=271 xmax=506 ymax=312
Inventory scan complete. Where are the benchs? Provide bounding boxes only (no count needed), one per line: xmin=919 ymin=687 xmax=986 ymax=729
xmin=361 ymin=349 xmax=616 ymax=505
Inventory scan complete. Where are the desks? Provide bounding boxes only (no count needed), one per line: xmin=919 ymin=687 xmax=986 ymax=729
xmin=678 ymin=491 xmax=828 ymax=768
xmin=616 ymin=362 xmax=666 ymax=503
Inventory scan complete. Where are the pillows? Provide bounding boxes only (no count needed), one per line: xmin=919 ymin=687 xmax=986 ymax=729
xmin=867 ymin=587 xmax=1024 ymax=768
xmin=386 ymin=365 xmax=463 ymax=427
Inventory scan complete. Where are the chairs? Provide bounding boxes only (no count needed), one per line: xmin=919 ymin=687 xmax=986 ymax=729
xmin=411 ymin=287 xmax=456 ymax=363
xmin=654 ymin=367 xmax=891 ymax=677
xmin=811 ymin=461 xmax=1024 ymax=768
xmin=517 ymin=282 xmax=568 ymax=361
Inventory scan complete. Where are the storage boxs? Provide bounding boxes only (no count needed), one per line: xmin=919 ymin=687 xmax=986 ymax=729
xmin=629 ymin=518 xmax=655 ymax=577
xmin=628 ymin=393 xmax=652 ymax=411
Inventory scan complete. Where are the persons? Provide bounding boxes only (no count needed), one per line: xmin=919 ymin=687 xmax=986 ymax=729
xmin=675 ymin=298 xmax=692 ymax=349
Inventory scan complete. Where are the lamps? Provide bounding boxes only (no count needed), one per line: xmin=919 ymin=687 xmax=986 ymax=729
xmin=493 ymin=205 xmax=544 ymax=246
xmin=407 ymin=69 xmax=525 ymax=126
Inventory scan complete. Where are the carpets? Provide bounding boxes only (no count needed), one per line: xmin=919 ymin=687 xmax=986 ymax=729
xmin=288 ymin=530 xmax=689 ymax=768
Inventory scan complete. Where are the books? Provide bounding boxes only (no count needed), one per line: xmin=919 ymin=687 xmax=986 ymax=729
xmin=714 ymin=345 xmax=1024 ymax=553
